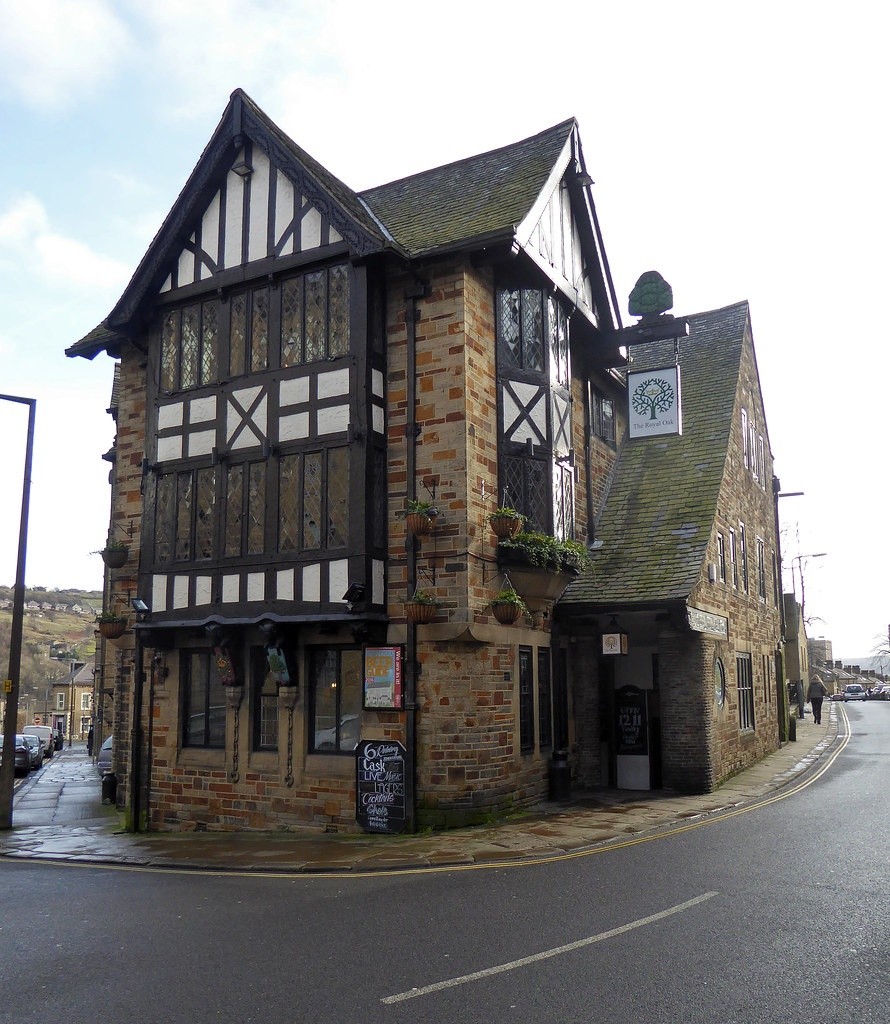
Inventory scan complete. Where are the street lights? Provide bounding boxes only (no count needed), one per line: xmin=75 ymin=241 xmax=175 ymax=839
xmin=792 ymin=553 xmax=827 ymax=594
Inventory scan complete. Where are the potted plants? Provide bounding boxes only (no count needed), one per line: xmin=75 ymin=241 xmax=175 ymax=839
xmin=392 ymin=497 xmax=441 ymax=537
xmin=486 ymin=509 xmax=596 ymax=578
xmin=87 ymin=527 xmax=132 ymax=570
xmin=95 ymin=595 xmax=127 ymax=639
xmin=396 ymin=572 xmax=439 ymax=625
xmin=489 ymin=574 xmax=534 ymax=627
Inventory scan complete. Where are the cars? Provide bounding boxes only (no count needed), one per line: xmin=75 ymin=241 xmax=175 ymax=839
xmin=0 ymin=736 xmax=33 ymax=776
xmin=98 ymin=734 xmax=114 ymax=775
xmin=315 ymin=714 xmax=358 ymax=752
xmin=53 ymin=728 xmax=63 ymax=751
xmin=18 ymin=735 xmax=46 ymax=771
xmin=829 ymin=684 xmax=890 ymax=703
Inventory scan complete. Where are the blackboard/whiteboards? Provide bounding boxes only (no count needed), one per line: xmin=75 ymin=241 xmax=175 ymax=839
xmin=355 ymin=738 xmax=408 ymax=835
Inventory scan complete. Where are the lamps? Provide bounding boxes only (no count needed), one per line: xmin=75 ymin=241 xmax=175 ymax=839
xmin=131 ymin=596 xmax=149 ymax=620
xmin=342 ymin=583 xmax=366 ymax=612
xmin=601 ymin=619 xmax=627 ymax=656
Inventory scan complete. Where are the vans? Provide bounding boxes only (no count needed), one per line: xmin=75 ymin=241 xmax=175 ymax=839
xmin=23 ymin=725 xmax=57 ymax=757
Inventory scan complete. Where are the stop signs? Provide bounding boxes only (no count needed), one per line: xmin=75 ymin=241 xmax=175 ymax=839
xmin=33 ymin=717 xmax=40 ymax=724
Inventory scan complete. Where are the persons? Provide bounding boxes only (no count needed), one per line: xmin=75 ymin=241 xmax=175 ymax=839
xmin=806 ymin=674 xmax=828 ymax=724
xmin=867 ymin=686 xmax=873 ymax=700
